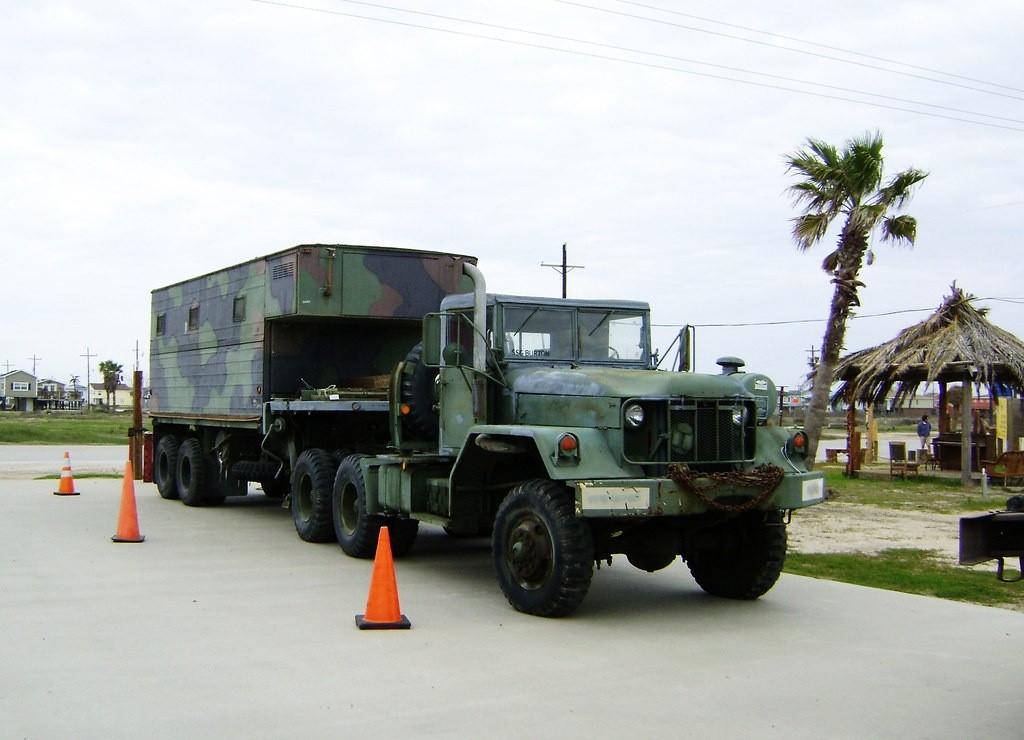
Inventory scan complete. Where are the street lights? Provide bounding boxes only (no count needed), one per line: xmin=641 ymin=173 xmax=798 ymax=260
xmin=776 ymin=385 xmax=789 ymax=425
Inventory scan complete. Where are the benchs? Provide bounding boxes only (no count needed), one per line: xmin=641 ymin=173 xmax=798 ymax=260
xmin=825 ymin=447 xmax=866 ymax=463
xmin=982 ymin=451 xmax=1024 ymax=487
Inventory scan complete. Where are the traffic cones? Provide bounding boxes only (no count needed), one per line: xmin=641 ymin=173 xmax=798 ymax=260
xmin=354 ymin=526 xmax=411 ymax=630
xmin=54 ymin=451 xmax=80 ymax=495
xmin=111 ymin=460 xmax=146 ymax=542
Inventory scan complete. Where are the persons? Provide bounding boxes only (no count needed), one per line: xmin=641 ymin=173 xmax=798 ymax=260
xmin=917 ymin=415 xmax=931 ymax=452
xmin=978 ymin=415 xmax=990 ymax=434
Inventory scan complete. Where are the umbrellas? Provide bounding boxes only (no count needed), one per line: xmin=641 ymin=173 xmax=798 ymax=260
xmin=807 ymin=287 xmax=1024 ymax=486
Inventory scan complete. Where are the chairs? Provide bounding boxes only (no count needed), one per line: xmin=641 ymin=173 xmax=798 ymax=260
xmin=889 ymin=441 xmax=919 ymax=481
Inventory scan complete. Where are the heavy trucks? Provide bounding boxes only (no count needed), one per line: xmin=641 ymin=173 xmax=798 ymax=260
xmin=145 ymin=244 xmax=827 ymax=617
